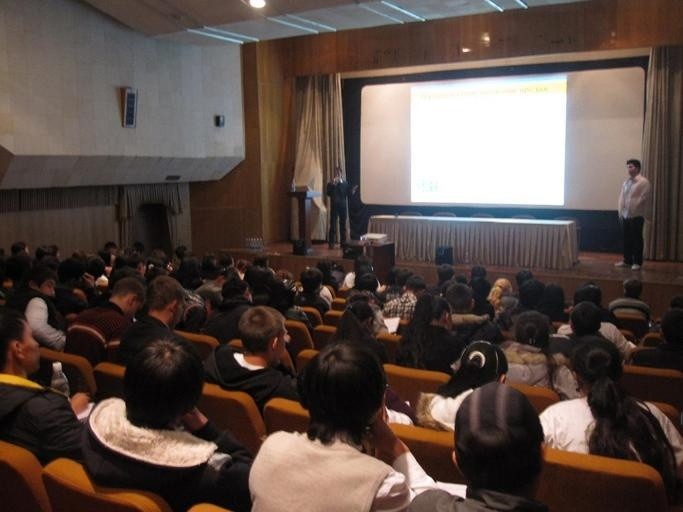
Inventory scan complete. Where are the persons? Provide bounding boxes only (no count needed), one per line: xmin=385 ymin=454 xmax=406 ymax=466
xmin=1 ymin=241 xmax=682 ymax=510
xmin=613 ymin=158 xmax=652 ymax=271
xmin=326 ymin=166 xmax=359 ymax=250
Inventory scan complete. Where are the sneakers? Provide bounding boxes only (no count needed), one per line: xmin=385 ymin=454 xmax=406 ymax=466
xmin=613 ymin=260 xmax=642 ymax=272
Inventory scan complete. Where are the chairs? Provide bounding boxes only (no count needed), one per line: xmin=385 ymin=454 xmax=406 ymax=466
xmin=400 ymin=211 xmax=578 ymax=220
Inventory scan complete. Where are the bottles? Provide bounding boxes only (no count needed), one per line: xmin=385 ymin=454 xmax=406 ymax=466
xmin=51 ymin=360 xmax=70 ymax=399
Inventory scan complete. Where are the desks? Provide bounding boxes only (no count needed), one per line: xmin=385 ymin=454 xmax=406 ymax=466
xmin=368 ymin=214 xmax=579 ymax=271
